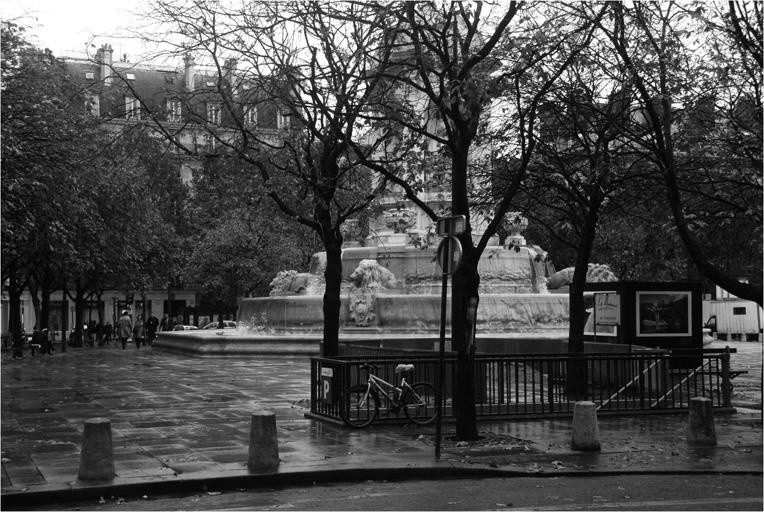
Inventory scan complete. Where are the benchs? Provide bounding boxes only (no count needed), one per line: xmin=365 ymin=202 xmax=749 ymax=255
xmin=20 ymin=330 xmax=65 ymax=357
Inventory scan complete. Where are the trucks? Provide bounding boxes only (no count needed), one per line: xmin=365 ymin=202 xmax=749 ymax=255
xmin=702 ymin=300 xmax=760 ymax=342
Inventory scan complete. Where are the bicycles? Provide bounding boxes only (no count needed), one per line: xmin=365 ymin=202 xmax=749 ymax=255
xmin=340 ymin=361 xmax=439 ymax=426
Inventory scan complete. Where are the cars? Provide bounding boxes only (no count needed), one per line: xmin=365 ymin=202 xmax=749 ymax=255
xmin=172 ymin=325 xmax=199 ymax=331
xmin=201 ymin=320 xmax=235 ymax=330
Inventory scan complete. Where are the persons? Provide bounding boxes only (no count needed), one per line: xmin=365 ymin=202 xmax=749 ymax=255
xmin=11 ymin=312 xmax=173 ymax=360
